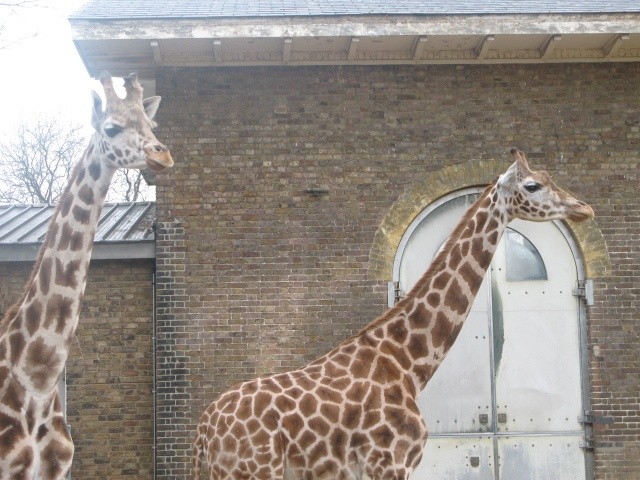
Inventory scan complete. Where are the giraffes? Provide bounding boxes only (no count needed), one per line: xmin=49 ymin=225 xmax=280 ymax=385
xmin=191 ymin=146 xmax=596 ymax=474
xmin=2 ymin=72 xmax=174 ymax=475
